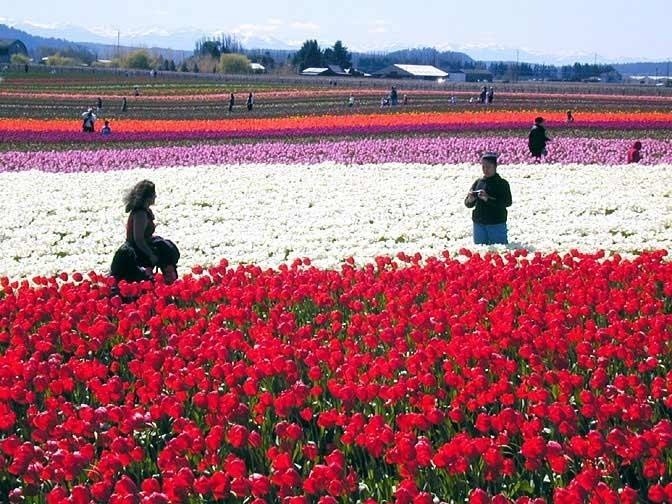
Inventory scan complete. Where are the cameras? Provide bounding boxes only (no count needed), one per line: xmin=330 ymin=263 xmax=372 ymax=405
xmin=472 ymin=190 xmax=480 ymax=198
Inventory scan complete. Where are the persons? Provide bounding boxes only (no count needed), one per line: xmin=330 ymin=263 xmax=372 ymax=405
xmin=81 ymin=98 xmax=112 ymax=137
xmin=134 ymin=87 xmax=140 ymax=96
xmin=121 ymin=98 xmax=128 ymax=111
xmin=628 ymin=139 xmax=642 ymax=164
xmin=247 ymin=92 xmax=254 ymax=111
xmin=390 ymin=87 xmax=397 ymax=105
xmin=478 ymin=86 xmax=494 ymax=104
xmin=528 ymin=118 xmax=552 ymax=158
xmin=348 ymin=95 xmax=355 ymax=107
xmin=464 ymin=152 xmax=512 ymax=245
xmin=229 ymin=92 xmax=234 ymax=111
xmin=125 ymin=180 xmax=179 ymax=282
xmin=566 ymin=111 xmax=578 ymax=137
